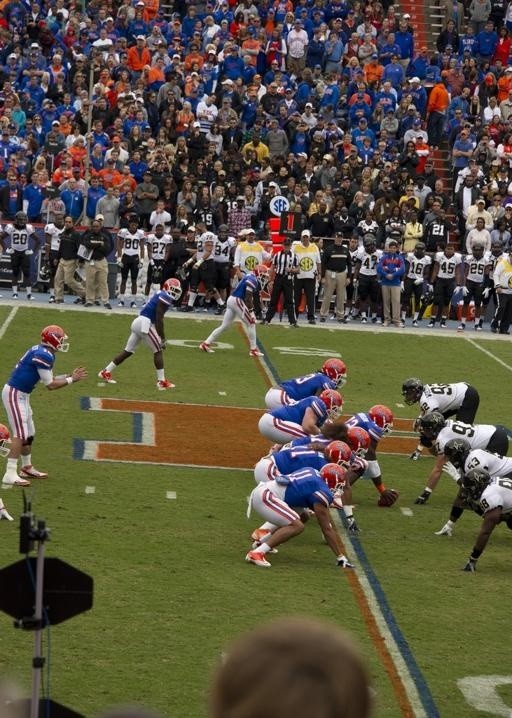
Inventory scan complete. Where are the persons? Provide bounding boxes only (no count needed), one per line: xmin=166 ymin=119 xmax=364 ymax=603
xmin=197 ymin=264 xmax=270 ymax=359
xmin=245 ymin=360 xmax=512 ymax=575
xmin=209 ymin=614 xmax=374 ymax=717
xmin=1 ymin=0 xmax=511 ymax=338
xmin=1 ymin=322 xmax=88 ymax=489
xmin=0 ymin=423 xmax=13 ymax=523
xmin=98 ymin=276 xmax=182 ymax=391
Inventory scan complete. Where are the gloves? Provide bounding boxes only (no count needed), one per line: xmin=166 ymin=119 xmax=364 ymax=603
xmin=6 ymin=248 xmax=15 ymax=254
xmin=115 ymin=261 xmax=165 ymax=273
xmin=434 ymin=521 xmax=455 ymax=538
xmin=24 ymin=249 xmax=33 ymax=256
xmin=463 ymin=557 xmax=477 ymax=574
xmin=248 ymin=308 xmax=257 ymax=323
xmin=261 ymin=308 xmax=268 ymax=319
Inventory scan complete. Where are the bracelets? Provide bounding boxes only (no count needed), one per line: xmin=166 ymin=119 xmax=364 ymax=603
xmin=65 ymin=376 xmax=73 ymax=385
xmin=54 ymin=373 xmax=67 ymax=380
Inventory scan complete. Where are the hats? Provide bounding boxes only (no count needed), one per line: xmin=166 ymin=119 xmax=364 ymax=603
xmin=95 ymin=214 xmax=104 ymax=221
xmin=188 ymin=226 xmax=343 ymax=238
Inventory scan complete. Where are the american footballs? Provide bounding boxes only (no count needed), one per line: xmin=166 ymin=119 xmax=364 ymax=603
xmin=378 ymin=488 xmax=400 ymax=506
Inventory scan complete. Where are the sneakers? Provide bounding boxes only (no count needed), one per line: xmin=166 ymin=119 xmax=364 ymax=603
xmin=249 ymin=346 xmax=265 ymax=357
xmin=199 ymin=342 xmax=216 ymax=353
xmin=98 ymin=369 xmax=118 ymax=384
xmin=156 ymin=380 xmax=176 ymax=390
xmin=19 ymin=465 xmax=48 ymax=479
xmin=245 ymin=491 xmax=355 ymax=568
xmin=2 ymin=471 xmax=31 ymax=487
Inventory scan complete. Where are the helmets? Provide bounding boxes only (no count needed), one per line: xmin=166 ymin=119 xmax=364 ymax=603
xmin=319 ymin=357 xmax=491 ymax=500
xmin=41 ymin=325 xmax=65 ymax=347
xmin=39 ymin=266 xmax=54 ymax=280
xmin=1 ymin=424 xmax=12 ymax=458
xmin=253 ymin=265 xmax=268 ymax=277
xmin=163 ymin=278 xmax=181 ymax=292
xmin=421 ymin=291 xmax=435 ymax=306
xmin=362 ymin=233 xmax=502 ymax=259
xmin=15 ymin=211 xmax=28 ymax=229
xmin=151 ymin=267 xmax=187 ymax=283
